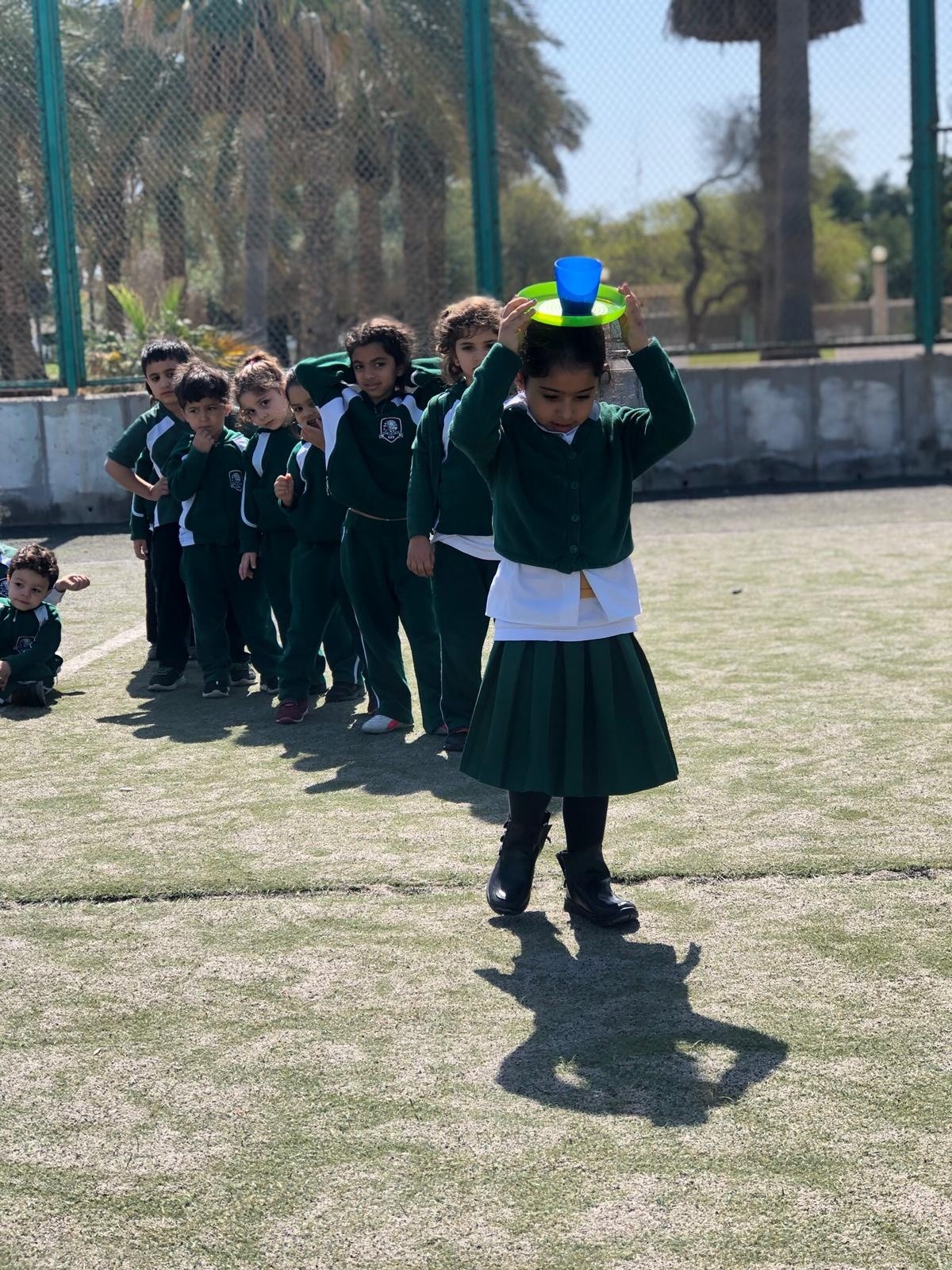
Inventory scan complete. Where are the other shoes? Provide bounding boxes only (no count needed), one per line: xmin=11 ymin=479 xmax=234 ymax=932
xmin=445 ymin=732 xmax=468 ymax=751
xmin=325 ymin=682 xmax=367 ymax=702
xmin=275 ymin=698 xmax=308 ymax=724
xmin=229 ymin=661 xmax=258 ymax=686
xmin=147 ymin=666 xmax=185 ymax=691
xmin=202 ymin=680 xmax=228 ymax=698
xmin=11 ymin=683 xmax=47 ymax=707
xmin=259 ymin=674 xmax=280 ymax=694
xmin=362 ymin=715 xmax=413 ymax=735
xmin=435 ymin=723 xmax=447 ymax=735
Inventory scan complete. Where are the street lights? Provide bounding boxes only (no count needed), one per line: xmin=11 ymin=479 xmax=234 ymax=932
xmin=870 ymin=245 xmax=890 ymax=337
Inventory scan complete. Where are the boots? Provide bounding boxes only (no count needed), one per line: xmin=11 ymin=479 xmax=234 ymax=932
xmin=486 ymin=809 xmax=553 ymax=916
xmin=556 ymin=849 xmax=639 ymax=928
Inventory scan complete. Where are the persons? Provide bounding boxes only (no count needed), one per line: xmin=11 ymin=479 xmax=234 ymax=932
xmin=103 ymin=338 xmax=284 ymax=698
xmin=296 ymin=314 xmax=453 ymax=734
xmin=450 ymin=281 xmax=680 ymax=926
xmin=273 ymin=352 xmax=377 ymax=724
xmin=235 ymin=349 xmax=329 ymax=696
xmin=407 ymin=296 xmax=517 ymax=752
xmin=0 ymin=543 xmax=90 ymax=707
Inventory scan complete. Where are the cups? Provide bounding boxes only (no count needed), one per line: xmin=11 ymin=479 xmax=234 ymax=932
xmin=554 ymin=256 xmax=603 ymax=315
